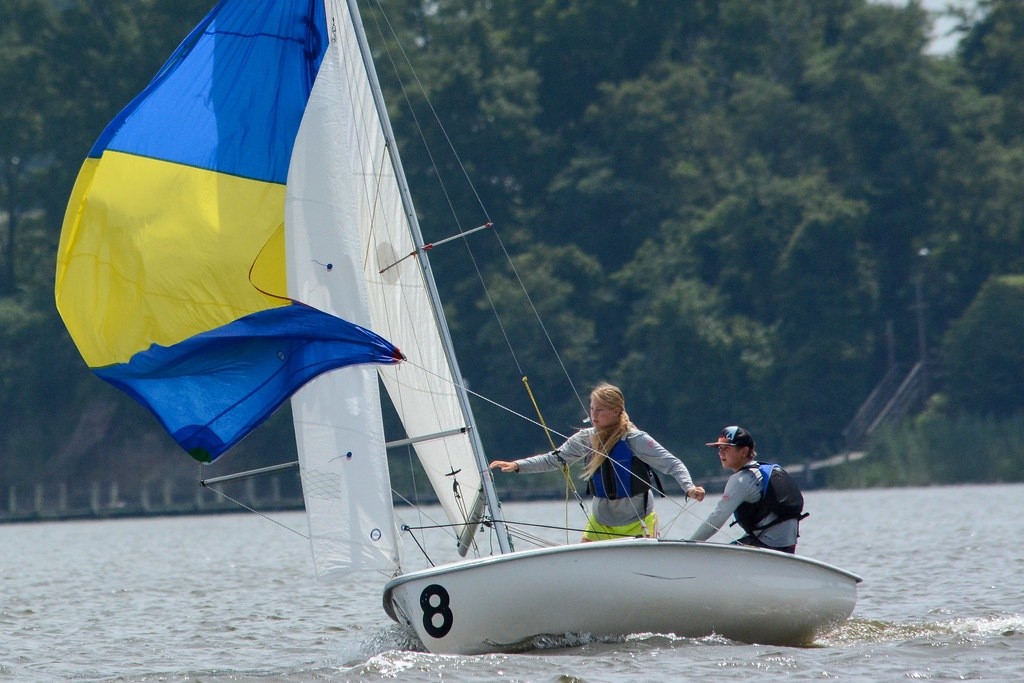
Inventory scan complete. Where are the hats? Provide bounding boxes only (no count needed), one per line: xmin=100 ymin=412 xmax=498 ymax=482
xmin=705 ymin=426 xmax=755 ymax=448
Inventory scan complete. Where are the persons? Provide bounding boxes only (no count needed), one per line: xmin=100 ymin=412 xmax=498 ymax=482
xmin=686 ymin=425 xmax=809 ymax=556
xmin=488 ymin=382 xmax=705 ymax=544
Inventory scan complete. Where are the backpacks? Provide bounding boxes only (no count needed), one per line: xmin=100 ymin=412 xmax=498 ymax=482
xmin=735 ymin=460 xmax=803 ymax=532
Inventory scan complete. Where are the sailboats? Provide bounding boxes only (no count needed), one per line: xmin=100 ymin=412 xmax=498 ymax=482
xmin=49 ymin=0 xmax=871 ymax=657
